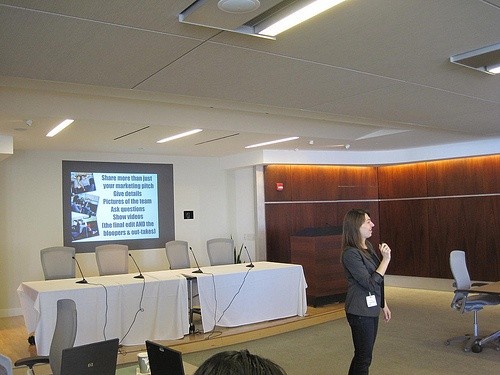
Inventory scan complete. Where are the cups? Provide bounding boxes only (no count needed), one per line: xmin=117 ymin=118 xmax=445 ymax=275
xmin=136 ymin=352 xmax=151 ymax=373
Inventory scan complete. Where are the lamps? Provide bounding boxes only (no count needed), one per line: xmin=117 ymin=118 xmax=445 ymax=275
xmin=449 ymin=41 xmax=500 ymax=75
xmin=177 ymin=0 xmax=342 ymax=40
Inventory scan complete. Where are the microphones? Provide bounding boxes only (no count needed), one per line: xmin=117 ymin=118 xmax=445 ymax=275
xmin=245 ymin=246 xmax=254 ymax=267
xmin=72 ymin=257 xmax=87 ymax=283
xmin=190 ymin=247 xmax=203 ymax=273
xmin=129 ymin=254 xmax=144 ymax=278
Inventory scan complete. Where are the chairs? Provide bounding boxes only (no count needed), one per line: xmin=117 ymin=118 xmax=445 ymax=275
xmin=40 ymin=246 xmax=76 ymax=280
xmin=14 ymin=299 xmax=77 ymax=375
xmin=443 ymin=250 xmax=500 ymax=353
xmin=95 ymin=243 xmax=129 ymax=277
xmin=165 ymin=240 xmax=190 ymax=270
xmin=207 ymin=238 xmax=235 ymax=266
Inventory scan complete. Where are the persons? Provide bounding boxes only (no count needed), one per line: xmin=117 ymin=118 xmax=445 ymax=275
xmin=72 ymin=219 xmax=98 ymax=240
xmin=71 ymin=175 xmax=90 ymax=193
xmin=340 ymin=207 xmax=392 ymax=375
xmin=72 ymin=198 xmax=94 ymax=214
xmin=192 ymin=351 xmax=287 ymax=375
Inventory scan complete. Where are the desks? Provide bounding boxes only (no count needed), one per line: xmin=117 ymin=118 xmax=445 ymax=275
xmin=466 ymin=281 xmax=500 ymax=352
xmin=177 ymin=261 xmax=307 ymax=333
xmin=18 ymin=270 xmax=188 ymax=356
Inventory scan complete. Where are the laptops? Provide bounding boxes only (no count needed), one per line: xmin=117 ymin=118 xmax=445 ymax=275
xmin=60 ymin=338 xmax=120 ymax=375
xmin=145 ymin=340 xmax=185 ymax=375
